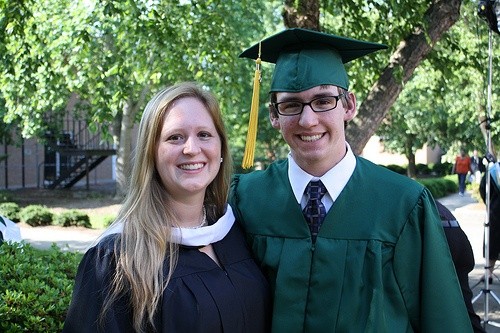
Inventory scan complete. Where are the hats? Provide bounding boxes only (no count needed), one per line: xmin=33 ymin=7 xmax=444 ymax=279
xmin=239 ymin=29 xmax=389 ymax=169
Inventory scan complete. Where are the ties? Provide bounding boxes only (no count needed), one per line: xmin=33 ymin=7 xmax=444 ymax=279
xmin=474 ymin=159 xmax=477 ymax=170
xmin=301 ymin=180 xmax=328 ymax=245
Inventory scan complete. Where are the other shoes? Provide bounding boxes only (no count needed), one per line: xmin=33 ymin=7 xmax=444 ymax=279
xmin=480 ymin=274 xmax=493 ymax=285
xmin=458 ymin=192 xmax=464 ymax=197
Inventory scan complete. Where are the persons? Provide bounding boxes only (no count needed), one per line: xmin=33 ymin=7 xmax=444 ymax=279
xmin=452 ymin=145 xmax=475 ymax=195
xmin=374 ymin=163 xmax=483 ymax=333
xmin=478 ymin=160 xmax=500 ymax=282
xmin=467 ymin=149 xmax=498 ymax=194
xmin=63 ymin=81 xmax=275 ymax=333
xmin=226 ymin=51 xmax=476 ymax=333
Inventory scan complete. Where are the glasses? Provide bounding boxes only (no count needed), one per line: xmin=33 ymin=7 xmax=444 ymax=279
xmin=273 ymin=94 xmax=345 ymax=115
